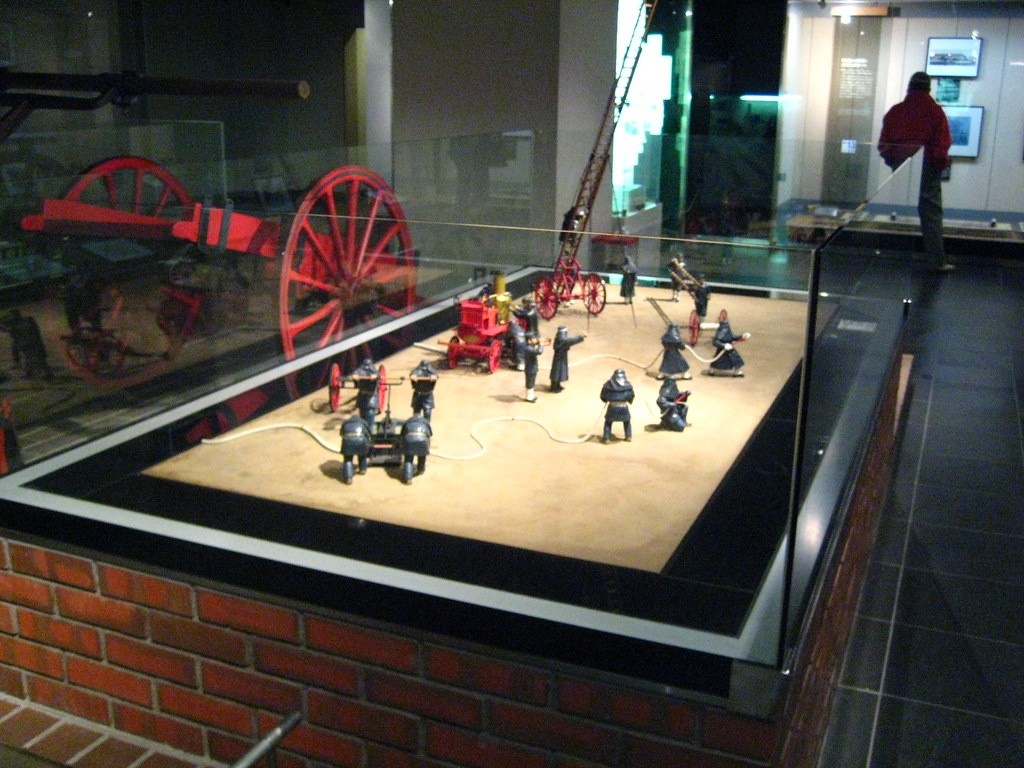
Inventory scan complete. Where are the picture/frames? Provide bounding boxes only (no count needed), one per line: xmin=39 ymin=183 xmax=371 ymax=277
xmin=938 ymin=104 xmax=984 ymax=158
xmin=925 ymin=36 xmax=984 ymax=78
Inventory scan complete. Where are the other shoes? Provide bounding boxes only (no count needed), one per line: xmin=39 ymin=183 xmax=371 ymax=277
xmin=933 ymin=265 xmax=955 ymax=273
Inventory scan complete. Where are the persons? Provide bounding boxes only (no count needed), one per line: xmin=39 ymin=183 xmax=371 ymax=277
xmin=878 ymin=71 xmax=956 ymax=270
xmin=351 ymin=249 xmax=752 ymax=444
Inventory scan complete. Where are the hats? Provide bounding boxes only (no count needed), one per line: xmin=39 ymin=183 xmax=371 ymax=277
xmin=909 ymin=72 xmax=931 ymax=91
